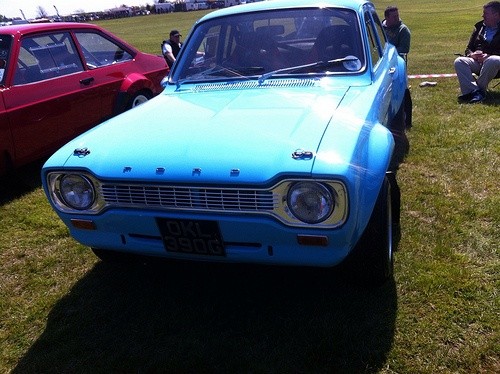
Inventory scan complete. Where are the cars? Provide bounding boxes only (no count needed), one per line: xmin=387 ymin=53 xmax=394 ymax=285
xmin=0 ymin=23 xmax=204 ymax=167
xmin=52 ymin=10 xmax=152 ymax=22
xmin=41 ymin=1 xmax=412 ymax=284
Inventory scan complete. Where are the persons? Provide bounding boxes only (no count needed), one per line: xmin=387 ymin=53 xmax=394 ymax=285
xmin=454 ymin=1 xmax=500 ymax=103
xmin=160 ymin=30 xmax=205 ymax=76
xmin=380 ymin=5 xmax=410 ymax=61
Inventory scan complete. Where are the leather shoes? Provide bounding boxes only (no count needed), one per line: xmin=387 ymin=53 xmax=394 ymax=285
xmin=458 ymin=91 xmax=487 ymax=103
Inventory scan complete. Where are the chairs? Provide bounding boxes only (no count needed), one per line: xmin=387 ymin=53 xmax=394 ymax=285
xmin=454 ymin=54 xmax=500 ymax=102
xmin=229 ymin=24 xmax=362 ymax=71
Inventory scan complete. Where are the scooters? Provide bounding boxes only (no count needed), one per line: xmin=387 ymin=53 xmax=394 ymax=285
xmin=168 ymin=54 xmax=205 ymax=74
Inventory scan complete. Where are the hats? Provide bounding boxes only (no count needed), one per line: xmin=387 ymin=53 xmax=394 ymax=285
xmin=170 ymin=30 xmax=182 ymax=37
